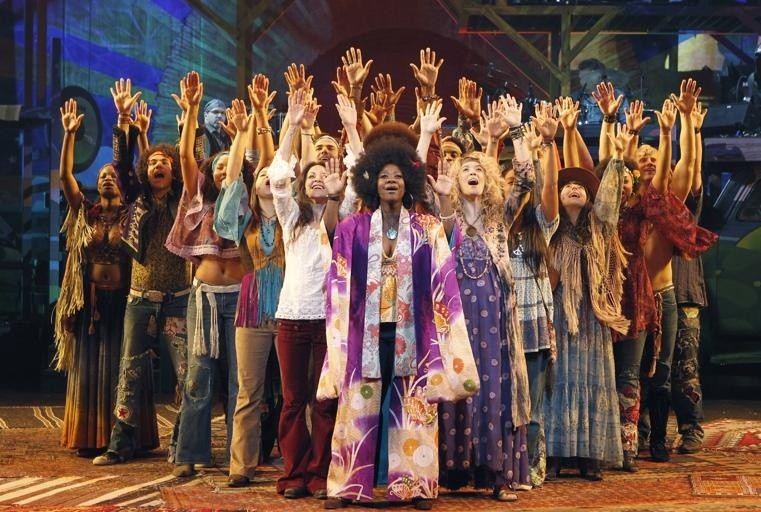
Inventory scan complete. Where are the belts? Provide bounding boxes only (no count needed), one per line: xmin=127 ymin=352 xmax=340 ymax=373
xmin=130 ymin=289 xmax=190 ymax=302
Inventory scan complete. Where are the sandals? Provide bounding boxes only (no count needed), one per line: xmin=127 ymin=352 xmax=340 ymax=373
xmin=494 ymin=488 xmax=517 ymax=500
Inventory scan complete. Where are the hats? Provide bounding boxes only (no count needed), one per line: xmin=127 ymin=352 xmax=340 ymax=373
xmin=558 ymin=168 xmax=600 ymax=199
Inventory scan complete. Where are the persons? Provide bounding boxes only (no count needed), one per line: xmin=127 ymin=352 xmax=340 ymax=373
xmin=51 ymin=46 xmax=720 ymax=511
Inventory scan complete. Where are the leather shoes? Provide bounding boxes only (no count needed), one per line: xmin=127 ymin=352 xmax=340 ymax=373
xmin=228 ymin=474 xmax=250 ymax=486
xmin=284 ymin=486 xmax=306 ymax=498
xmin=173 ymin=463 xmax=193 ymax=477
xmin=650 ymin=444 xmax=669 ymax=462
xmin=93 ymin=452 xmax=121 ymax=465
xmin=414 ymin=497 xmax=433 ymax=510
xmin=678 ymin=429 xmax=703 ymax=454
xmin=324 ymin=497 xmax=349 ymax=509
xmin=314 ymin=489 xmax=326 ymax=499
